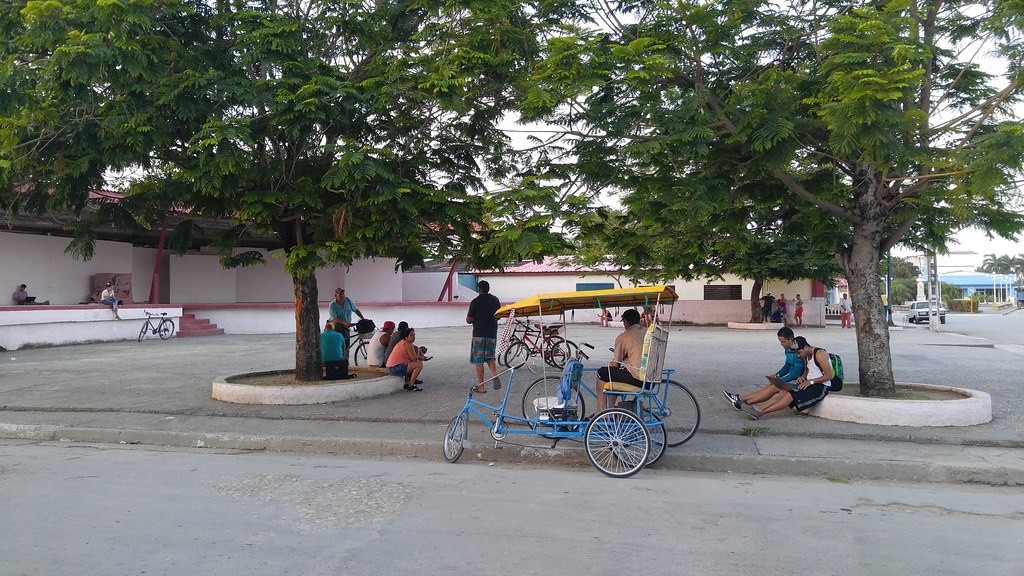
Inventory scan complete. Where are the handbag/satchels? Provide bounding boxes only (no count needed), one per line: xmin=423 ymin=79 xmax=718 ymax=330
xmin=356 ymin=319 xmax=376 ymax=333
xmin=324 ymin=360 xmax=357 ymax=379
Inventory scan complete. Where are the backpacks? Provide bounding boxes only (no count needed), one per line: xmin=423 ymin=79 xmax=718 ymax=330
xmin=814 ymin=348 xmax=844 ymax=391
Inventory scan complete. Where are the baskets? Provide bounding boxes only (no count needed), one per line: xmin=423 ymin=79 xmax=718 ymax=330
xmin=358 ymin=327 xmax=379 ymax=339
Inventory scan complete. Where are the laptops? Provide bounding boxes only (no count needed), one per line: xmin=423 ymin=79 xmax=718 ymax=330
xmin=765 ymin=376 xmax=803 ymax=392
xmin=20 ymin=297 xmax=36 ymax=303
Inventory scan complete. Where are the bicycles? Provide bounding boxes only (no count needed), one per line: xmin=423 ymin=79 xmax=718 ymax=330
xmin=138 ymin=309 xmax=175 ymax=343
xmin=497 ymin=320 xmax=582 ymax=370
xmin=521 ymin=341 xmax=702 ymax=448
xmin=339 ymin=321 xmax=375 ymax=366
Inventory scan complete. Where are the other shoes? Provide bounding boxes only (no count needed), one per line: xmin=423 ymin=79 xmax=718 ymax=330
xmin=112 ymin=309 xmax=118 ymax=312
xmin=587 ymin=414 xmax=615 ymax=422
xmin=741 ymin=403 xmax=763 ymax=420
xmin=115 ymin=316 xmax=122 ymax=320
xmin=414 ymin=378 xmax=424 ymax=384
xmin=404 ymin=384 xmax=423 ymax=392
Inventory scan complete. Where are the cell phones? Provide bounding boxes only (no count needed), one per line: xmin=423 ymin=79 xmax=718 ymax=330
xmin=609 ymin=348 xmax=614 ymax=352
xmin=424 ymin=356 xmax=434 ymax=361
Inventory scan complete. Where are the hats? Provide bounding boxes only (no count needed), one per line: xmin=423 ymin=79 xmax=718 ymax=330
xmin=620 ymin=309 xmax=640 ymax=324
xmin=766 ymin=291 xmax=771 ymax=295
xmin=787 ymin=336 xmax=807 ymax=353
xmin=380 ymin=321 xmax=396 ymax=330
xmin=325 ymin=321 xmax=335 ymax=330
xmin=476 ymin=280 xmax=490 ymax=292
xmin=335 ymin=288 xmax=345 ymax=293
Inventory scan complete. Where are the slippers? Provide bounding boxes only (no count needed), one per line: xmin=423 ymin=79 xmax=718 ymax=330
xmin=472 ymin=386 xmax=486 ymax=393
xmin=493 ymin=376 xmax=501 ymax=390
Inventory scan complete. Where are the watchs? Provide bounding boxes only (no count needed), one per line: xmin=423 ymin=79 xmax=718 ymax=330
xmin=810 ymin=379 xmax=815 ymax=385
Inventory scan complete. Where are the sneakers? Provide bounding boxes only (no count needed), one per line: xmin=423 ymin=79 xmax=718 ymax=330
xmin=724 ymin=390 xmax=747 ymax=410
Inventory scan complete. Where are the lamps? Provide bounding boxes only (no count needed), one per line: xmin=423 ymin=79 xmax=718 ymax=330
xmin=453 ymin=295 xmax=459 ymax=300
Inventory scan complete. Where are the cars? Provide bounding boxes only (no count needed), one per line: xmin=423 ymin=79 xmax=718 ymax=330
xmin=907 ymin=301 xmax=946 ymax=325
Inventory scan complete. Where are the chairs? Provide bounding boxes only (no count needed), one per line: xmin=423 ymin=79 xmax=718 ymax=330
xmin=533 ymin=357 xmax=583 ymax=411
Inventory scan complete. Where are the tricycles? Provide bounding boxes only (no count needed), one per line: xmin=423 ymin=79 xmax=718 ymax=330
xmin=443 ymin=285 xmax=679 ymax=477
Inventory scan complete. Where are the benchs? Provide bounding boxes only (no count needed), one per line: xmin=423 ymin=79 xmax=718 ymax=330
xmin=603 ymin=323 xmax=669 ymax=394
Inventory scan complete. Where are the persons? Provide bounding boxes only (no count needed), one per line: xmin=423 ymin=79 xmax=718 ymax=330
xmin=320 ymin=319 xmax=346 ymax=365
xmin=367 ymin=321 xmax=433 ymax=392
xmin=582 ymin=309 xmax=656 ymax=422
xmin=100 ymin=282 xmax=122 ymax=321
xmin=792 ymin=294 xmax=804 ymax=327
xmin=759 ymin=291 xmax=788 ymax=327
xmin=597 ymin=307 xmax=613 ymax=327
xmin=13 ymin=284 xmax=50 ymax=305
xmin=723 ymin=326 xmax=834 ymax=421
xmin=640 ymin=305 xmax=659 ymax=326
xmin=466 ymin=280 xmax=501 ymax=393
xmin=880 ymin=292 xmax=888 ymax=317
xmin=837 ymin=292 xmax=852 ymax=329
xmin=329 ymin=288 xmax=365 ymax=366
